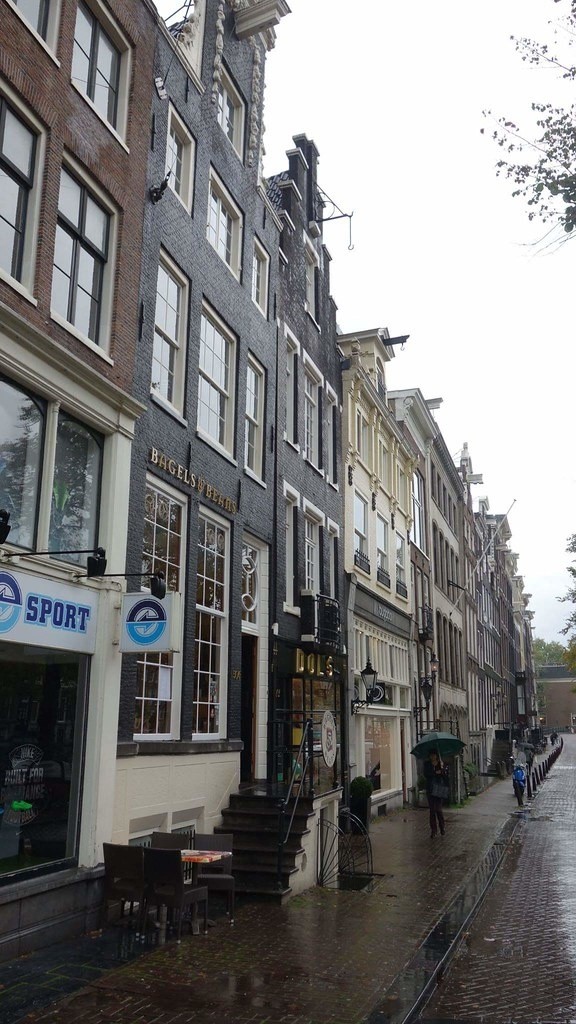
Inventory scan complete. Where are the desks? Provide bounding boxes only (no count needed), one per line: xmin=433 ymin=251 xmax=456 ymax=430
xmin=181 ymin=849 xmax=232 ymax=934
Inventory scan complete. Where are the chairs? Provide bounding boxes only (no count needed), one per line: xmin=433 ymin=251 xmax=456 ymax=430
xmin=194 ymin=833 xmax=235 ymax=934
xmin=150 ymin=831 xmax=190 ymax=867
xmin=100 ymin=843 xmax=144 ymax=932
xmin=144 ymin=847 xmax=207 ymax=944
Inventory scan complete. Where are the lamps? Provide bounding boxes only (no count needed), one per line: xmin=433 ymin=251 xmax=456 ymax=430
xmin=351 ymin=656 xmax=378 ymax=715
xmin=4 ymin=547 xmax=107 ymax=578
xmin=413 ymin=676 xmax=432 ymax=718
xmin=429 ymin=652 xmax=439 ymax=682
xmin=74 ymin=571 xmax=167 ymax=600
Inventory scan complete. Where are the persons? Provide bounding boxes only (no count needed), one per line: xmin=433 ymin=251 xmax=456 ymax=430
xmin=526 ymin=749 xmax=532 ymax=775
xmin=423 ymin=748 xmax=449 ymax=839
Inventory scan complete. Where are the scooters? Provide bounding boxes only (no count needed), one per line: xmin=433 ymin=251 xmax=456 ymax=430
xmin=514 ymin=762 xmax=528 ymax=796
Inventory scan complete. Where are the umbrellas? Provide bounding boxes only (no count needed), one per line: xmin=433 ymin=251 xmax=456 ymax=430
xmin=520 ymin=743 xmax=534 ymax=751
xmin=410 ymin=731 xmax=468 ymax=768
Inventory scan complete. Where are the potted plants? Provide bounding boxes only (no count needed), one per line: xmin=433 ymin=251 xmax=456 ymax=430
xmin=349 ymin=776 xmax=373 ymax=835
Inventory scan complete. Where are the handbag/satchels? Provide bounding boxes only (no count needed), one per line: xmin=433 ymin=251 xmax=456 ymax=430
xmin=431 ymin=776 xmax=449 ymax=799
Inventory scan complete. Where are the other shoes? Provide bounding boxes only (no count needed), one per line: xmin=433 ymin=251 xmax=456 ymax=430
xmin=440 ymin=827 xmax=445 ymax=835
xmin=430 ymin=832 xmax=437 ymax=838
